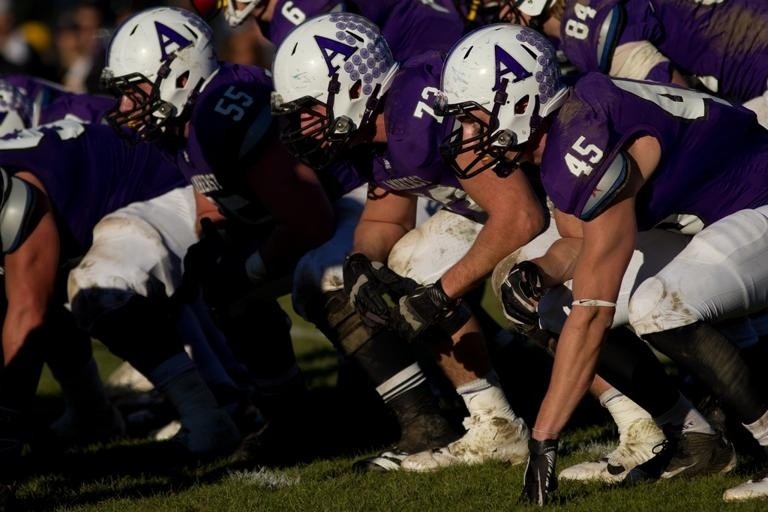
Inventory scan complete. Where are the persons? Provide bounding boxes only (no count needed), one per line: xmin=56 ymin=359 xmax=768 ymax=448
xmin=0 ymin=1 xmax=768 ymax=506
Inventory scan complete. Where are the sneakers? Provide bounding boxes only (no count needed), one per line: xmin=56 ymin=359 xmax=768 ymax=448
xmin=558 ymin=418 xmax=768 ymax=502
xmin=353 ymin=413 xmax=530 ymax=474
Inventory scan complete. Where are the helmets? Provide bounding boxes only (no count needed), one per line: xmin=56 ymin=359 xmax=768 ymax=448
xmin=457 ymin=0 xmax=556 ymax=27
xmin=269 ymin=11 xmax=402 ymax=191
xmin=217 ymin=0 xmax=261 ymax=28
xmin=433 ymin=23 xmax=571 ymax=181
xmin=100 ymin=7 xmax=221 ymax=153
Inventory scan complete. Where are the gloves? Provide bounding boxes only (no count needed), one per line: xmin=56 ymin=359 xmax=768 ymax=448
xmin=496 ymin=260 xmax=545 ymax=327
xmin=342 ymin=252 xmax=464 ymax=347
xmin=516 ymin=439 xmax=563 ymax=509
